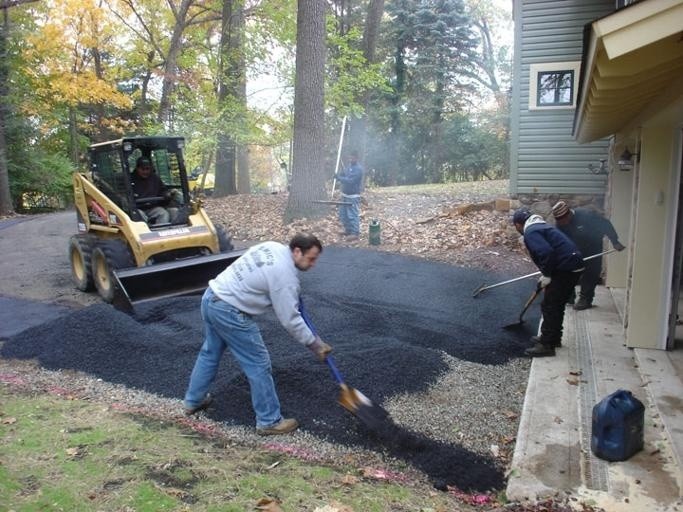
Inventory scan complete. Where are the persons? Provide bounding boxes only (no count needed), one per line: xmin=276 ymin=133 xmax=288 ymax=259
xmin=183 ymin=232 xmax=332 ymax=435
xmin=513 ymin=208 xmax=586 ymax=356
xmin=552 ymin=202 xmax=625 ymax=309
xmin=333 ymin=151 xmax=363 ymax=241
xmin=132 ymin=157 xmax=171 ymax=224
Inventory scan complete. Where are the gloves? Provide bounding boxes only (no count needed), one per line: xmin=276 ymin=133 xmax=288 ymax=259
xmin=536 ymin=275 xmax=551 ymax=289
xmin=305 ymin=334 xmax=332 ymax=365
xmin=613 ymin=241 xmax=625 ymax=252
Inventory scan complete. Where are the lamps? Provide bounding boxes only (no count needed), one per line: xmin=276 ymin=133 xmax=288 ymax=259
xmin=588 ymin=158 xmax=612 ymax=189
xmin=616 ymin=145 xmax=639 ymax=172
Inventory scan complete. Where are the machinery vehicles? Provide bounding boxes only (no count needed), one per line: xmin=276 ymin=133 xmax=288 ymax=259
xmin=64 ymin=136 xmax=254 ymax=316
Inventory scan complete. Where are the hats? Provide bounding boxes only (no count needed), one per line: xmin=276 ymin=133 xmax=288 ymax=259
xmin=551 ymin=200 xmax=570 ymax=220
xmin=135 ymin=156 xmax=153 ymax=167
xmin=512 ymin=207 xmax=531 ymax=224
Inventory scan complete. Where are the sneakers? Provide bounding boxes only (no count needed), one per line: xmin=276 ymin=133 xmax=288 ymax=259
xmin=183 ymin=391 xmax=212 ymax=415
xmin=524 ymin=329 xmax=562 ymax=357
xmin=566 ymin=294 xmax=576 ymax=304
xmin=572 ymin=294 xmax=594 ymax=310
xmin=337 ymin=230 xmax=360 ymax=242
xmin=255 ymin=417 xmax=299 ymax=434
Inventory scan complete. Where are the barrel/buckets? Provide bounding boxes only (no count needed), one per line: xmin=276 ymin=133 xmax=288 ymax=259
xmin=591 ymin=389 xmax=645 ymax=461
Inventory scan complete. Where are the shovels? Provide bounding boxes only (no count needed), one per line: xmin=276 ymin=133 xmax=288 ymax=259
xmin=503 ymin=282 xmax=546 ymax=330
xmin=299 ymin=299 xmax=391 ymax=430
